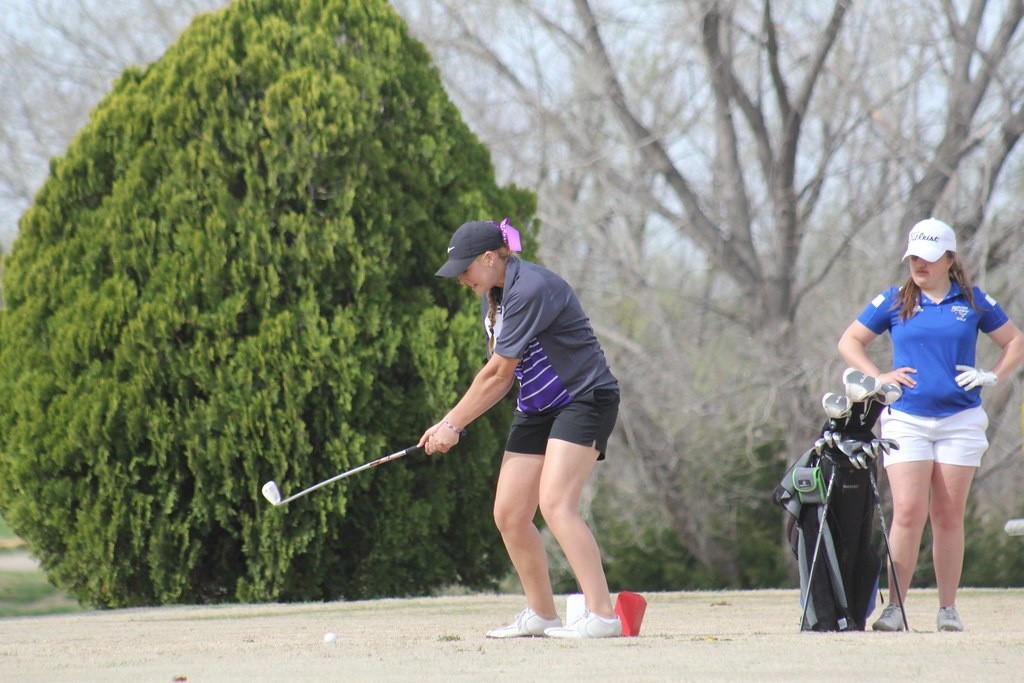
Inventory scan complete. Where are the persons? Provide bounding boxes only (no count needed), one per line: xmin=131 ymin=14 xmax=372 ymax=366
xmin=417 ymin=218 xmax=622 ymax=639
xmin=838 ymin=218 xmax=1024 ymax=632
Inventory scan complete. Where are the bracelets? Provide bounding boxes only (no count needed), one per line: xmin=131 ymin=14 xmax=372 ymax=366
xmin=444 ymin=418 xmax=462 ymax=433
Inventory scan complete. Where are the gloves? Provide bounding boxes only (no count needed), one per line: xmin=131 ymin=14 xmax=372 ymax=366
xmin=955 ymin=364 xmax=997 ymax=392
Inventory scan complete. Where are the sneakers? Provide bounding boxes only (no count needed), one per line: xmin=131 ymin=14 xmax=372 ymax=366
xmin=486 ymin=609 xmax=560 ymax=638
xmin=936 ymin=604 xmax=962 ymax=631
xmin=873 ymin=604 xmax=904 ymax=632
xmin=544 ymin=610 xmax=623 ymax=639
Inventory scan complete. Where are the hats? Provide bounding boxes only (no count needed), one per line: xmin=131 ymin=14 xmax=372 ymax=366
xmin=901 ymin=217 xmax=957 ymax=263
xmin=435 ymin=222 xmax=506 ymax=277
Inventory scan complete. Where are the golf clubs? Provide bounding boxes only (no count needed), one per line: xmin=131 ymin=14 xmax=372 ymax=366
xmin=261 ymin=445 xmax=421 ymax=505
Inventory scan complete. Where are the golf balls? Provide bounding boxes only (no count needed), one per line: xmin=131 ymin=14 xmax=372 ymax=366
xmin=811 ymin=367 xmax=904 ymax=470
xmin=325 ymin=632 xmax=336 ymax=644
